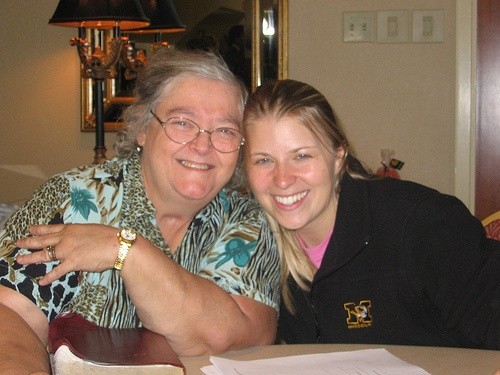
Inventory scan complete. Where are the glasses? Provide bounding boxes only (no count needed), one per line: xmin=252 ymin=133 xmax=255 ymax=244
xmin=148 ymin=107 xmax=245 ymax=153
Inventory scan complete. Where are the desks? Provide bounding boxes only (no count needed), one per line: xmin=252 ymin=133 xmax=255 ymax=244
xmin=178 ymin=343 xmax=500 ymax=375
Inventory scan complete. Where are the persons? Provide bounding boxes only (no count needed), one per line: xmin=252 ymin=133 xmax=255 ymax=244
xmin=241 ymin=79 xmax=500 ymax=350
xmin=0 ymin=48 xmax=282 ymax=375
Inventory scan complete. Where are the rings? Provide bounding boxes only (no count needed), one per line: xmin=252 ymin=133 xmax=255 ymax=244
xmin=46 ymin=246 xmax=57 ymax=260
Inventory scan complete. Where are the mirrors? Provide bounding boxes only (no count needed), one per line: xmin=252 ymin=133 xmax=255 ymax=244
xmin=81 ymin=0 xmax=289 ymax=131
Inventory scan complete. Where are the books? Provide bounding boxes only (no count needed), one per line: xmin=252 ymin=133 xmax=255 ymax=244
xmin=47 ymin=311 xmax=186 ymax=375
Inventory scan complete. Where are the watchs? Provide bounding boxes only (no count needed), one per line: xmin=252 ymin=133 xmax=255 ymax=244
xmin=114 ymin=229 xmax=137 ymax=271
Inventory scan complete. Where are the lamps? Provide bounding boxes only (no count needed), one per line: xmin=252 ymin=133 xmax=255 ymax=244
xmin=48 ymin=0 xmax=151 ymax=166
xmin=121 ymin=0 xmax=187 ymax=42
